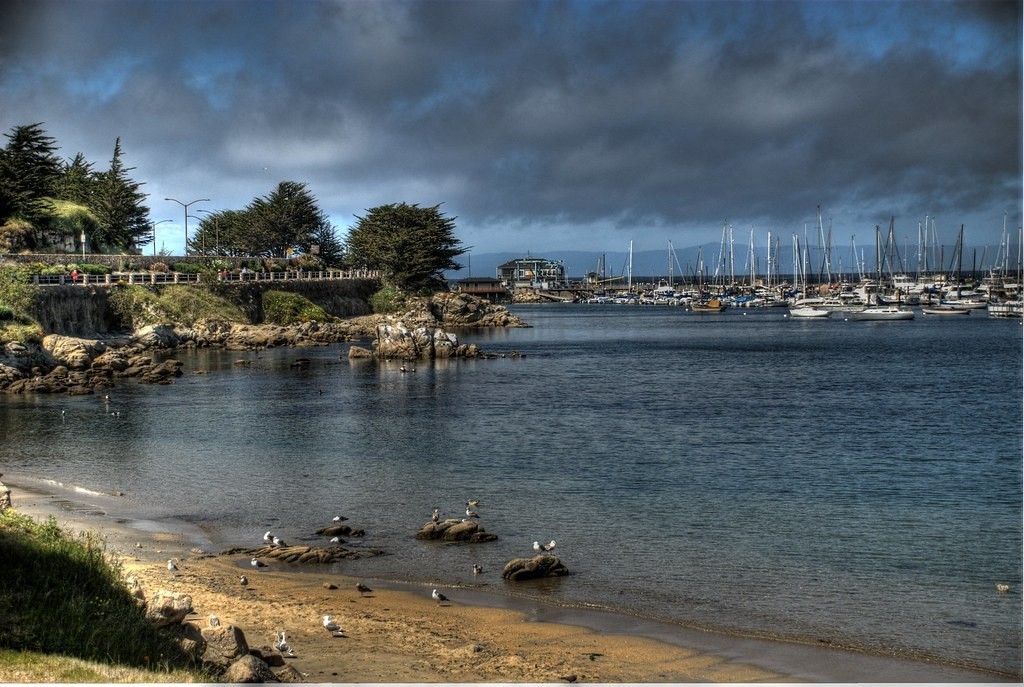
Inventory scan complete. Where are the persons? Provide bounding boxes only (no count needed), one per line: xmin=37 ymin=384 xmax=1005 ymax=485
xmin=241 ymin=267 xmax=247 ymax=281
xmin=223 ymin=269 xmax=229 ymax=281
xmin=72 ymin=270 xmax=78 ymax=285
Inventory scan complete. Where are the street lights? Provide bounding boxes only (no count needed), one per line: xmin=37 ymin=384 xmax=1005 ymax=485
xmin=187 ymin=215 xmax=205 ymax=256
xmin=164 ymin=197 xmax=210 ymax=256
xmin=196 ymin=209 xmax=219 ymax=256
xmin=153 ymin=220 xmax=173 ymax=255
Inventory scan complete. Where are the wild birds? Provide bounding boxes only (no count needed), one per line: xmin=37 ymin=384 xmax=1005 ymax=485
xmin=264 ymin=530 xmax=287 ymax=549
xmin=330 ymin=536 xmax=349 ymax=548
xmin=166 ymin=559 xmax=180 ymax=578
xmin=332 ymin=515 xmax=349 ymax=525
xmin=238 ymin=575 xmax=248 ymax=588
xmin=271 ymin=630 xmax=295 ymax=658
xmin=136 ymin=541 xmax=143 ymax=551
xmin=432 ymin=589 xmax=451 ymax=607
xmin=35 ymin=373 xmax=121 ymax=417
xmin=557 ymin=674 xmax=578 ymax=683
xmin=473 ymin=564 xmax=483 ymax=574
xmin=321 ymin=615 xmax=349 ymax=638
xmin=208 ymin=611 xmax=220 ymax=627
xmin=431 ymin=499 xmax=481 ymax=522
xmin=250 ymin=558 xmax=269 ymax=571
xmin=532 ymin=540 xmax=557 ymax=557
xmin=356 ymin=582 xmax=374 ymax=598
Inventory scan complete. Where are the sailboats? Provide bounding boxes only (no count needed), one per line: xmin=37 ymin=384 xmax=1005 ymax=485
xmin=562 ymin=204 xmax=1023 ymax=322
